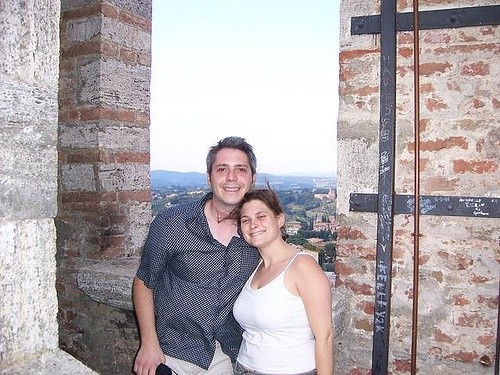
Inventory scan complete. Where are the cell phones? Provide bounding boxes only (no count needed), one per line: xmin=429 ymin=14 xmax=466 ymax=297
xmin=155 ymin=363 xmax=172 ymax=375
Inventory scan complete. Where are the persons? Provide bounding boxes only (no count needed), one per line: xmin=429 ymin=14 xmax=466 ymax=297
xmin=231 ymin=189 xmax=335 ymax=374
xmin=131 ymin=136 xmax=261 ymax=375
